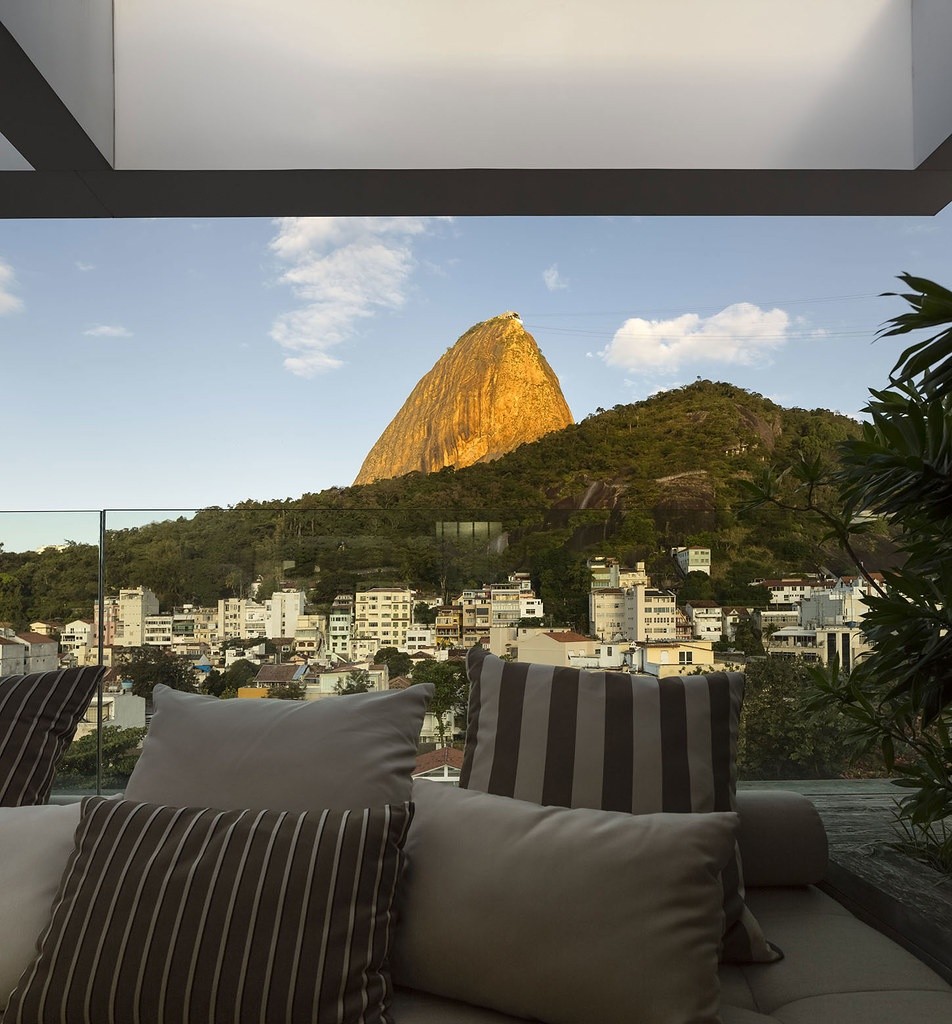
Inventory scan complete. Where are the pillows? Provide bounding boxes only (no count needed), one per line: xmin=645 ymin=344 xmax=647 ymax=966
xmin=0 ymin=665 xmax=106 ymax=808
xmin=124 ymin=683 xmax=437 ymax=810
xmin=0 ymin=797 xmax=415 ymax=1024
xmin=0 ymin=804 xmax=81 ymax=962
xmin=393 ymin=780 xmax=741 ymax=1024
xmin=461 ymin=645 xmax=783 ymax=961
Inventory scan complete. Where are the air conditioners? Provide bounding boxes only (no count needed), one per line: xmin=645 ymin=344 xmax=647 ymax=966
xmin=282 ymin=645 xmax=290 ymax=652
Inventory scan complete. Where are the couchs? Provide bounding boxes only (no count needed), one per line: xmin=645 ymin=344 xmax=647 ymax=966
xmin=386 ymin=789 xmax=952 ymax=1024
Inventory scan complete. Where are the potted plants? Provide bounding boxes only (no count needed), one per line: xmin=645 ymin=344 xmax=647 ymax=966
xmin=812 ymin=263 xmax=952 ymax=979
